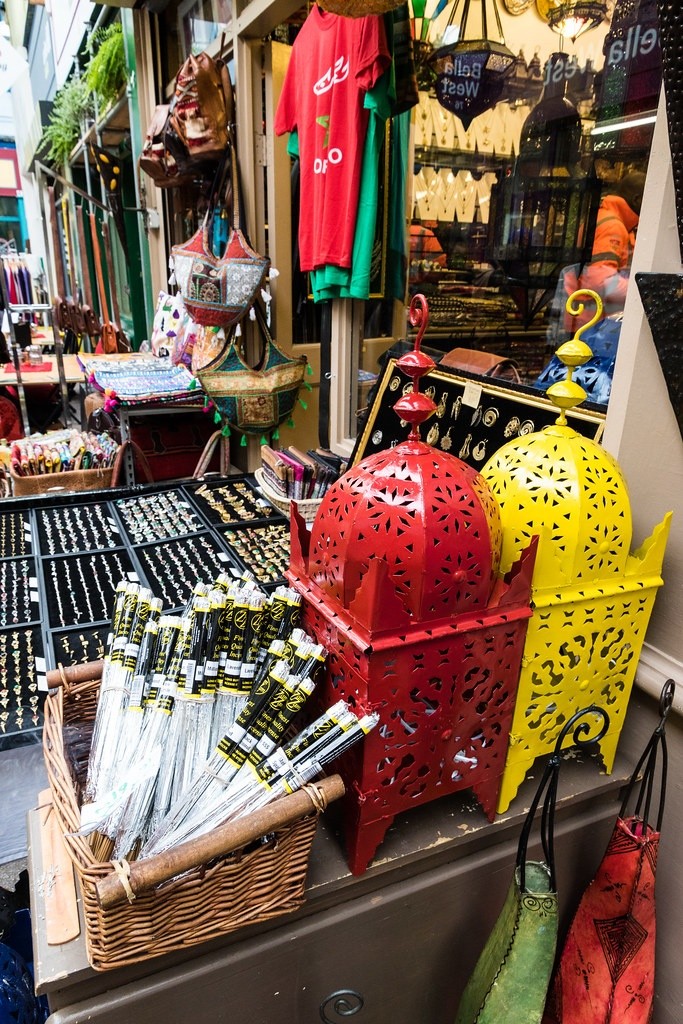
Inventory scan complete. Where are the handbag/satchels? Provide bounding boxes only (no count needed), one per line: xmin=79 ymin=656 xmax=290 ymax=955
xmin=54 ymin=297 xmax=132 ymax=354
xmin=151 ymin=145 xmax=313 ymax=446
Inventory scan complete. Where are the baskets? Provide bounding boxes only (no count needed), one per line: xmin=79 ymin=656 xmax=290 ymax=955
xmin=254 ymin=467 xmax=322 ymax=523
xmin=42 ymin=661 xmax=346 ymax=974
xmin=9 ymin=463 xmax=114 ymax=497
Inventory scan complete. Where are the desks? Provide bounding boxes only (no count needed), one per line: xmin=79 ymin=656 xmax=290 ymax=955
xmin=0 ymin=352 xmax=151 ymax=437
xmin=28 ymin=745 xmax=647 ymax=1024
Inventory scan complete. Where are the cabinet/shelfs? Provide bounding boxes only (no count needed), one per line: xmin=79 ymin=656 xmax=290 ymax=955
xmin=396 ymin=51 xmax=596 ymax=374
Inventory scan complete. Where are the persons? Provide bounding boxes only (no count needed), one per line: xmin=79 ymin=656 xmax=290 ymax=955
xmin=407 ymin=221 xmax=447 ymax=279
xmin=564 ymin=172 xmax=647 ymax=341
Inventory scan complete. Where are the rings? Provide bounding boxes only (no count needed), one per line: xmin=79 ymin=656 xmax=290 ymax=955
xmin=0 ymin=483 xmax=291 ymax=732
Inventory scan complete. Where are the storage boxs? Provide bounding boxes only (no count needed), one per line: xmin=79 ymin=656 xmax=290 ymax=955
xmin=0 ymin=344 xmax=677 ymax=973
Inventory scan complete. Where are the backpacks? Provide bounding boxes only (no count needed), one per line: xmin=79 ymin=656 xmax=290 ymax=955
xmin=139 ymin=104 xmax=190 ymax=188
xmin=170 ymin=31 xmax=232 ymax=170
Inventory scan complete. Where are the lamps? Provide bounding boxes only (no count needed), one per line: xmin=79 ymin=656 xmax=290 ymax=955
xmin=546 ymin=0 xmax=610 ymax=43
